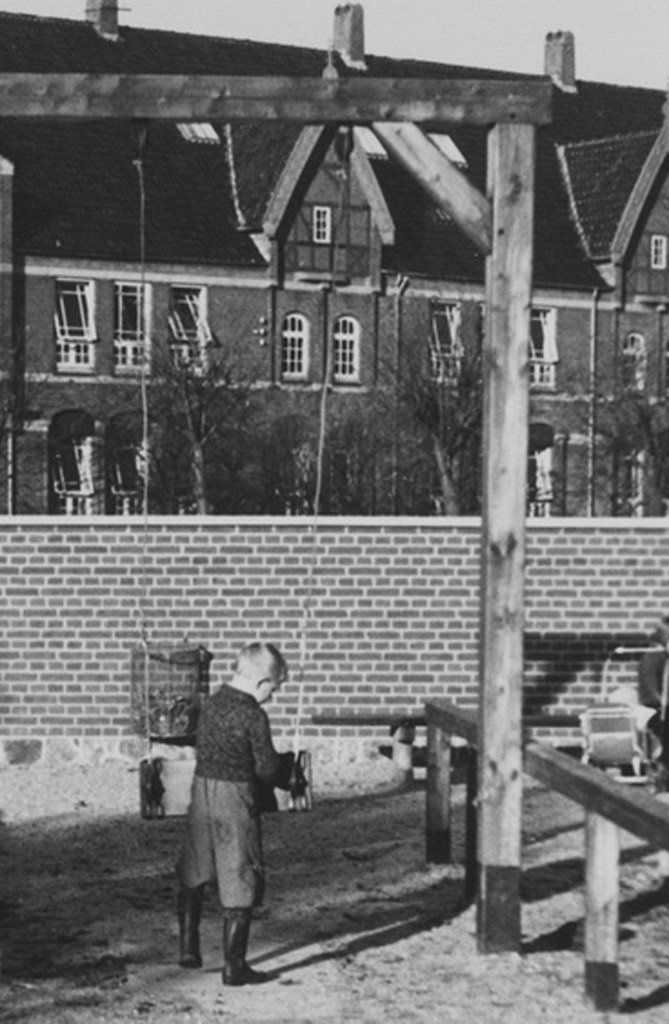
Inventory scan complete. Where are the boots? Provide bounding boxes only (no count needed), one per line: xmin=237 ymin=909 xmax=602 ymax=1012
xmin=223 ymin=908 xmax=269 ymax=985
xmin=179 ymin=884 xmax=202 ymax=968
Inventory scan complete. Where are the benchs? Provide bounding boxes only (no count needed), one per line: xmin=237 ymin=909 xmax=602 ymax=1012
xmin=312 ymin=715 xmax=590 ymax=783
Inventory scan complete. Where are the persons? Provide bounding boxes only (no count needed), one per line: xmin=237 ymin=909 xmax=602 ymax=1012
xmin=637 ymin=617 xmax=669 ymax=793
xmin=176 ymin=644 xmax=310 ymax=987
